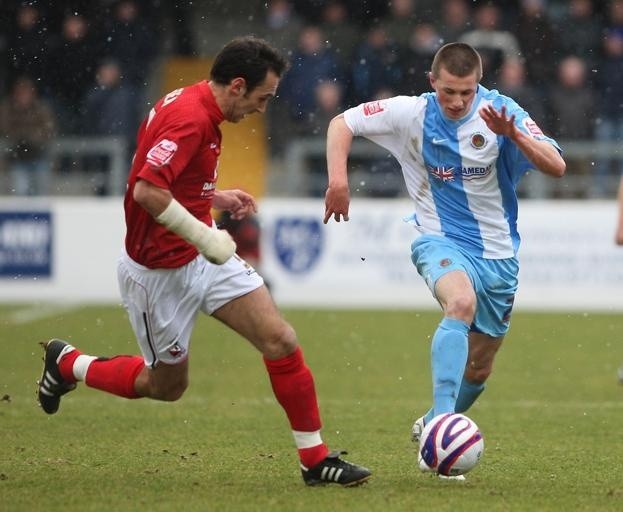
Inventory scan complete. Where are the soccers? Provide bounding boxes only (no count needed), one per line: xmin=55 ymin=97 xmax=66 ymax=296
xmin=419 ymin=413 xmax=484 ymax=476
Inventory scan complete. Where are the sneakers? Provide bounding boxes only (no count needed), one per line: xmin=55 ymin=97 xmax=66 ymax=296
xmin=417 ymin=447 xmax=467 ymax=481
xmin=300 ymin=450 xmax=371 ymax=486
xmin=36 ymin=337 xmax=76 ymax=415
xmin=412 ymin=415 xmax=425 ymax=442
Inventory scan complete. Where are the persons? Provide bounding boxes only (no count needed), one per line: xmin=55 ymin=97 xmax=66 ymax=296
xmin=322 ymin=41 xmax=568 ymax=481
xmin=0 ymin=3 xmax=621 ymax=199
xmin=32 ymin=38 xmax=373 ymax=488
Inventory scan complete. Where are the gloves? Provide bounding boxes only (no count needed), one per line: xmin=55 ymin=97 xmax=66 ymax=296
xmin=155 ymin=197 xmax=238 ymax=265
